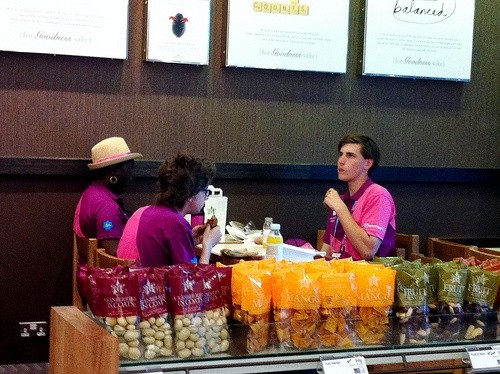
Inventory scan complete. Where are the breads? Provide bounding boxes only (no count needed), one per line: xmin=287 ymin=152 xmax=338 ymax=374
xmin=254 ymin=236 xmax=264 ymax=244
xmin=206 ymin=215 xmax=218 ymax=228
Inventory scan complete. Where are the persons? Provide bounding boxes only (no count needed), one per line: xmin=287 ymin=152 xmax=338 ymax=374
xmin=285 ymin=133 xmax=398 ymax=261
xmin=72 ymin=136 xmax=143 ymax=256
xmin=117 ymin=153 xmax=223 ymax=269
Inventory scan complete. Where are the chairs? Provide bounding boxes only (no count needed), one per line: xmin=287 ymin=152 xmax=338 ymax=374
xmin=72 ymin=231 xmax=135 ymax=305
xmin=317 ymin=230 xmax=500 ymax=263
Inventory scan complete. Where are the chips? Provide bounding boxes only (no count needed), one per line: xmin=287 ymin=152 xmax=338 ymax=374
xmin=233 ymin=307 xmax=390 ymax=348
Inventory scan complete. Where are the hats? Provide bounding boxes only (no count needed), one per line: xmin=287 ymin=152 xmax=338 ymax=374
xmin=86 ymin=136 xmax=147 ymax=170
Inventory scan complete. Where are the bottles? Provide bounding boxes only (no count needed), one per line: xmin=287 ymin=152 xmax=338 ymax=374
xmin=266 ymin=224 xmax=284 ymax=262
xmin=262 ymin=217 xmax=273 ymax=248
xmin=191 ymin=202 xmax=205 ymax=229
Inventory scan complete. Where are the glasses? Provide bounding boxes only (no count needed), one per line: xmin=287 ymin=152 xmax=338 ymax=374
xmin=199 ymin=189 xmax=213 ymax=197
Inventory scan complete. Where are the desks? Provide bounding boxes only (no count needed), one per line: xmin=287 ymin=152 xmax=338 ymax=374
xmin=194 ymin=234 xmax=330 ymax=265
